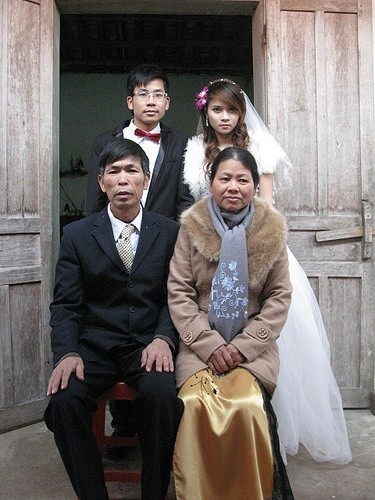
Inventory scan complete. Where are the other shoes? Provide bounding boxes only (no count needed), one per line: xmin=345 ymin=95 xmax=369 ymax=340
xmin=109 ymin=428 xmax=136 ymax=462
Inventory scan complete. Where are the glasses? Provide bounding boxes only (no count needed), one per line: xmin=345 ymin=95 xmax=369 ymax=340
xmin=133 ymin=91 xmax=167 ymax=99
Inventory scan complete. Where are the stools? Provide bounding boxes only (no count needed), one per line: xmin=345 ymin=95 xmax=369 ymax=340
xmin=92 ymin=382 xmax=142 ymax=481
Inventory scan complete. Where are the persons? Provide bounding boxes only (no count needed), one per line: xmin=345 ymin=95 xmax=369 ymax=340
xmin=182 ymin=79 xmax=352 ymax=465
xmin=164 ymin=147 xmax=296 ymax=500
xmin=44 ymin=139 xmax=182 ymax=500
xmin=83 ymin=65 xmax=195 ymax=459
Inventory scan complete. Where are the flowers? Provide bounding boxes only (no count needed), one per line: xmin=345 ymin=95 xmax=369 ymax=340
xmin=194 ymin=86 xmax=209 ymax=109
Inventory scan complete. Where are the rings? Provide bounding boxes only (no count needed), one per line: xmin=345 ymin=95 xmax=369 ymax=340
xmin=162 ymin=357 xmax=168 ymax=361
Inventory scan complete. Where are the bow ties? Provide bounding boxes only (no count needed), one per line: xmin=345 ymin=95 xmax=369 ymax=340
xmin=134 ymin=128 xmax=161 ymax=143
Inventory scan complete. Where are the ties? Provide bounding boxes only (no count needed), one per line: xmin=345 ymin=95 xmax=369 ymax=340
xmin=117 ymin=224 xmax=137 ymax=273
